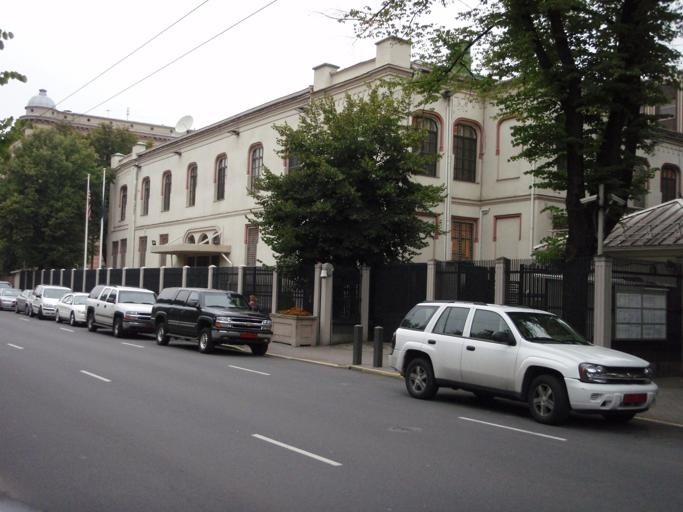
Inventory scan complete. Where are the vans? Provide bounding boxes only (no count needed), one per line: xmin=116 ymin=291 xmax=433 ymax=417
xmin=0 ymin=281 xmax=13 ymax=289
xmin=30 ymin=284 xmax=72 ymax=320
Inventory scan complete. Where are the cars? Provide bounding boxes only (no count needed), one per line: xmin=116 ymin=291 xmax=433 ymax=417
xmin=16 ymin=289 xmax=33 ymax=315
xmin=53 ymin=292 xmax=89 ymax=325
xmin=0 ymin=288 xmax=19 ymax=311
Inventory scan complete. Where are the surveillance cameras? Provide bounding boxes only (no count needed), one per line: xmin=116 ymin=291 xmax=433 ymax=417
xmin=580 ymin=191 xmax=600 ymax=204
xmin=609 ymin=194 xmax=627 ymax=208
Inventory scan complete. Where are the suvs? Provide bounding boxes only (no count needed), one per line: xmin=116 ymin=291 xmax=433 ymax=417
xmin=389 ymin=299 xmax=657 ymax=425
xmin=86 ymin=282 xmax=156 ymax=338
xmin=150 ymin=285 xmax=272 ymax=358
